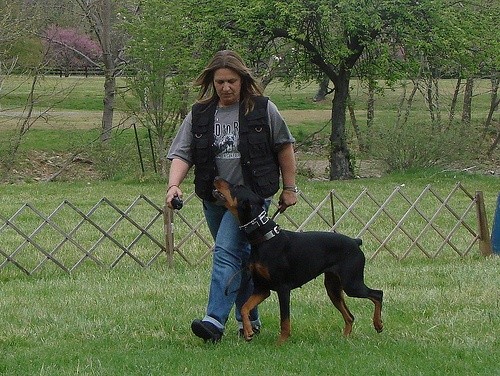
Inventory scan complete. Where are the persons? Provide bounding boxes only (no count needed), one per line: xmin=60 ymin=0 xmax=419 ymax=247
xmin=166 ymin=50 xmax=298 ymax=342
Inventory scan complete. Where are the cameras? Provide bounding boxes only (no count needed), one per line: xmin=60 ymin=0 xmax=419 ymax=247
xmin=172 ymin=196 xmax=183 ymax=210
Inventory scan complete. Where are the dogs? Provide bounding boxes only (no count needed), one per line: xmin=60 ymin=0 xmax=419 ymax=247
xmin=212 ymin=177 xmax=383 ymax=345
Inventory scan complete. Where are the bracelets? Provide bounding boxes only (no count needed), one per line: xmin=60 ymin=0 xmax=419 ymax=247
xmin=283 ymin=185 xmax=298 ymax=193
xmin=166 ymin=185 xmax=178 ymax=193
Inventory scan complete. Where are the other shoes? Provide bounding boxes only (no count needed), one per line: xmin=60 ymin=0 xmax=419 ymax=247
xmin=238 ymin=328 xmax=261 ymax=340
xmin=192 ymin=318 xmax=223 ymax=345
